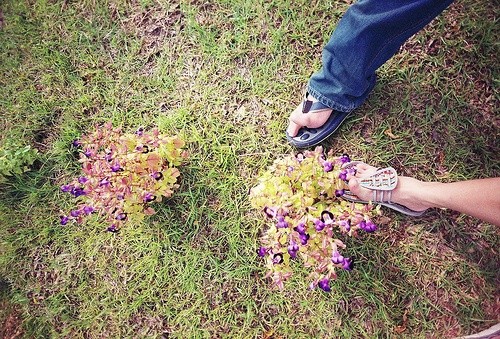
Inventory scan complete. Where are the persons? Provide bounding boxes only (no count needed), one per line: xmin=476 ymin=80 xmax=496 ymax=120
xmin=336 ymin=161 xmax=500 ymax=339
xmin=286 ymin=0 xmax=453 ymax=148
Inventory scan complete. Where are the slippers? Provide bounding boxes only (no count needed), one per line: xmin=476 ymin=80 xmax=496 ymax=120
xmin=284 ymin=68 xmax=379 ymax=148
xmin=338 ymin=162 xmax=428 ymax=217
